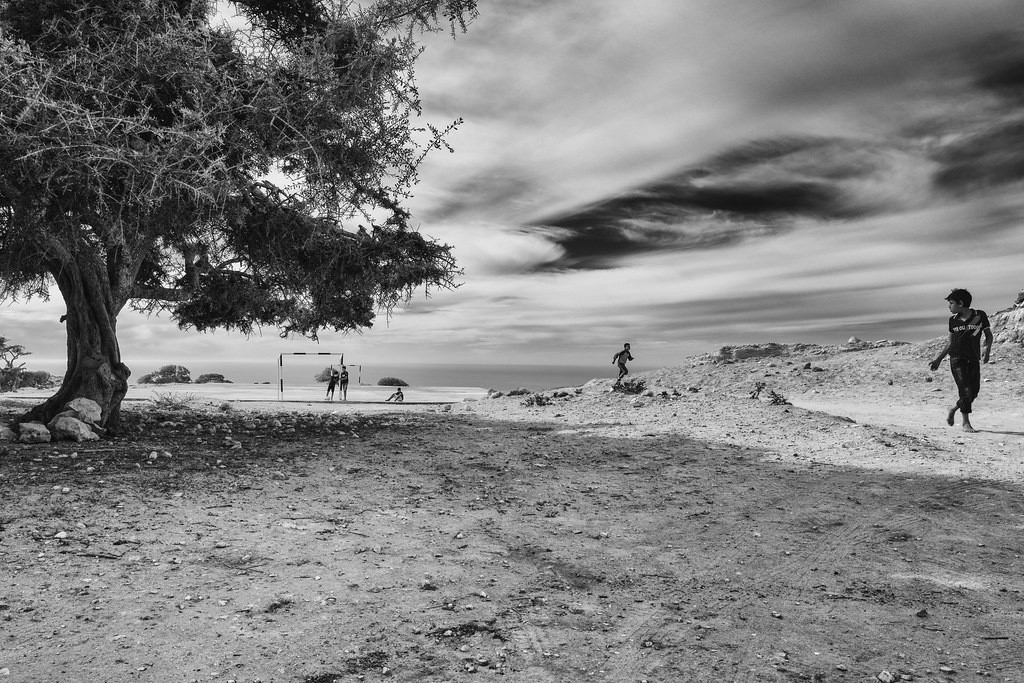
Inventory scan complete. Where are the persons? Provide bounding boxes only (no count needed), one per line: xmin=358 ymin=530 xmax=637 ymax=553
xmin=385 ymin=388 xmax=404 ymax=403
xmin=612 ymin=343 xmax=634 ymax=382
xmin=929 ymin=287 xmax=994 ymax=432
xmin=324 ymin=371 xmax=340 ymax=401
xmin=340 ymin=366 xmax=349 ymax=401
